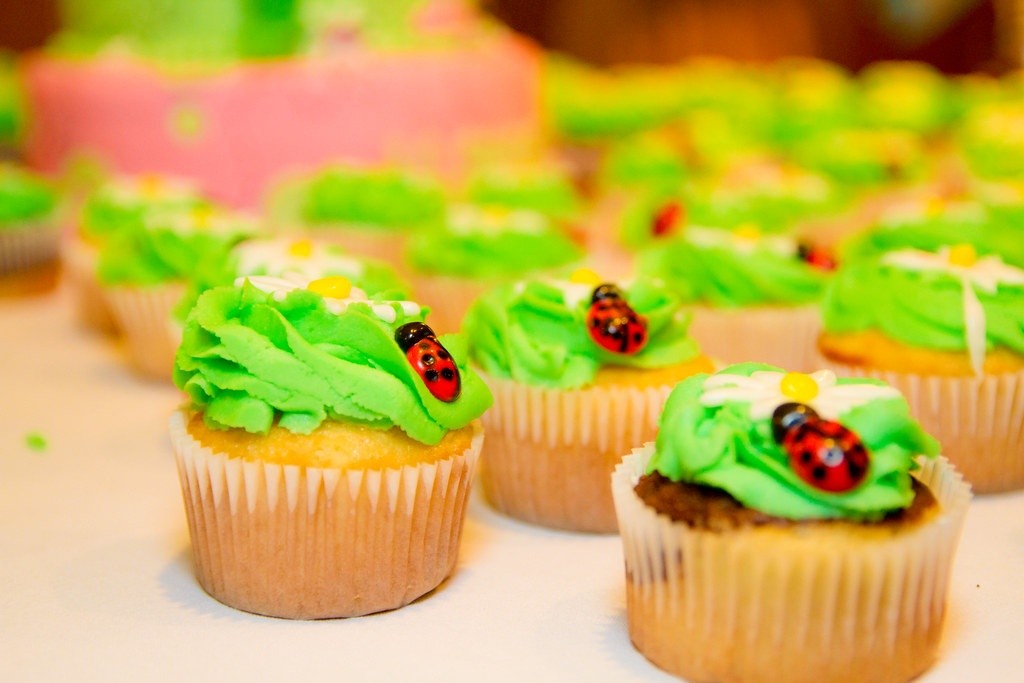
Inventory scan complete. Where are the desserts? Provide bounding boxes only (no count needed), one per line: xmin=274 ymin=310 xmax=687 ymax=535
xmin=0 ymin=0 xmax=1024 ymax=683
